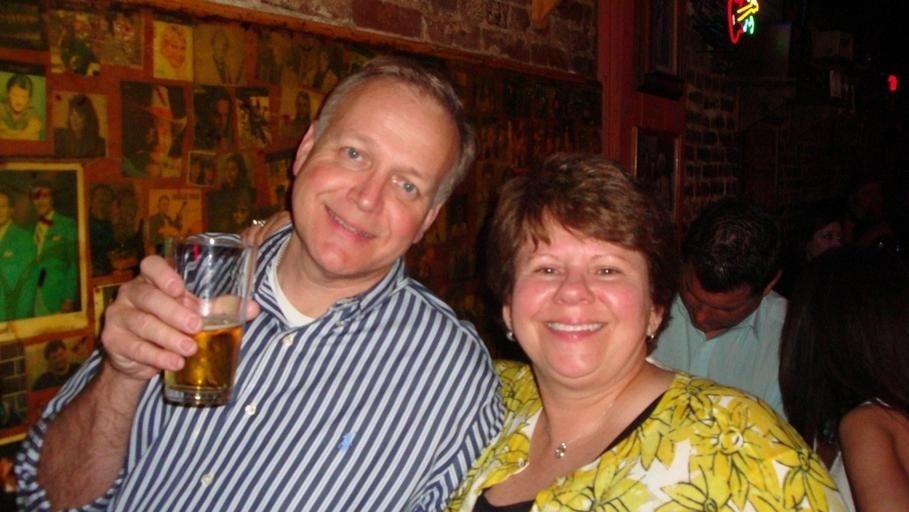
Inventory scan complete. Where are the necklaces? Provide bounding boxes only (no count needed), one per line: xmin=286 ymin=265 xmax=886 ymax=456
xmin=543 ymin=403 xmax=613 ymax=457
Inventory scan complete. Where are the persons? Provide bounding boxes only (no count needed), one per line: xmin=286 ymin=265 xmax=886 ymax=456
xmin=1 ymin=2 xmax=601 ymax=391
xmin=648 ymin=198 xmax=908 ymax=512
xmin=14 ymin=63 xmax=506 ymax=511
xmin=446 ymin=152 xmax=846 ymax=512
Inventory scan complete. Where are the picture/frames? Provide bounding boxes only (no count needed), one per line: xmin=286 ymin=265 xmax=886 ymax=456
xmin=88 ymin=274 xmax=135 ymax=358
xmin=0 ymin=156 xmax=95 ymax=348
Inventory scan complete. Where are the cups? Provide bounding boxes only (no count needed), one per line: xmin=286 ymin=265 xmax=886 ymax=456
xmin=162 ymin=236 xmax=252 ymax=407
